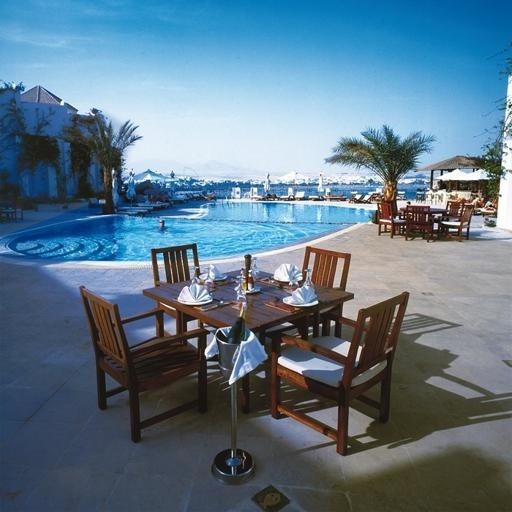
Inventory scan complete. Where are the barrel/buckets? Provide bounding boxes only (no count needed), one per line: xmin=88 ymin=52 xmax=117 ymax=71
xmin=215 ymin=327 xmax=255 ymax=379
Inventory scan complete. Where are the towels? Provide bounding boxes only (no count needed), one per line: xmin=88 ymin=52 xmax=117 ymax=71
xmin=289 ymin=285 xmax=318 ymax=305
xmin=178 ymin=283 xmax=211 ymax=303
xmin=206 ymin=264 xmax=225 ymax=280
xmin=204 ymin=327 xmax=268 ymax=386
xmin=274 ymin=264 xmax=303 ymax=287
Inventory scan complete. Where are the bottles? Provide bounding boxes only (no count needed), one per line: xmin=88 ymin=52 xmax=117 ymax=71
xmin=192 ymin=267 xmax=201 ymax=285
xmin=241 ymin=269 xmax=254 ymax=291
xmin=226 ymin=302 xmax=248 ymax=345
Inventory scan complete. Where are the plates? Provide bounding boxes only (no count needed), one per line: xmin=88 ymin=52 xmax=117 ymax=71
xmin=272 ymin=273 xmax=303 ymax=283
xmin=199 ymin=272 xmax=228 ymax=281
xmin=282 ymin=295 xmax=320 ymax=308
xmin=177 ymin=296 xmax=213 ymax=306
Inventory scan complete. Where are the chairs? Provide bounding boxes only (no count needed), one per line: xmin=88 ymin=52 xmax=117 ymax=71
xmin=270 ymin=291 xmax=408 ymax=458
xmin=110 ymin=186 xmax=204 ymax=215
xmin=291 ymin=246 xmax=351 ymax=337
xmin=230 ymin=187 xmax=496 ymax=241
xmin=78 ymin=285 xmax=211 ymax=442
xmin=151 ymin=244 xmax=202 ymax=334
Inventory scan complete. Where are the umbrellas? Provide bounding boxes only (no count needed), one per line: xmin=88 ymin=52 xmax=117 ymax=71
xmin=317 ymin=174 xmax=324 ymax=196
xmin=458 ymin=168 xmax=499 ymax=193
xmin=436 ymin=167 xmax=464 ymax=192
xmin=263 ymin=172 xmax=270 ymax=195
